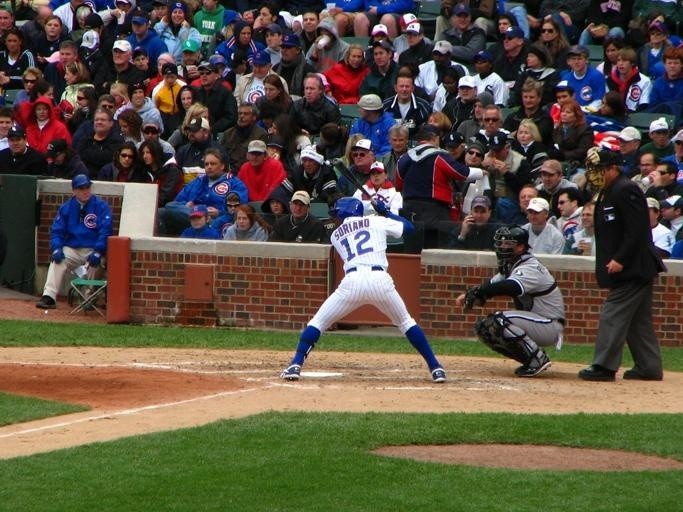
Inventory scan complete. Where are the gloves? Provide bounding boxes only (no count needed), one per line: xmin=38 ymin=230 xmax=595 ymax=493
xmin=87 ymin=249 xmax=102 ymax=268
xmin=50 ymin=247 xmax=65 ymax=264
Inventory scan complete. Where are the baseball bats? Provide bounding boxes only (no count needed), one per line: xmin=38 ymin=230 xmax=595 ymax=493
xmin=334 ymin=159 xmax=377 ymax=206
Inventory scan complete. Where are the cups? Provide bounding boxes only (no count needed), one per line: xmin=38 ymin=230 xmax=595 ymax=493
xmin=642 ymin=175 xmax=654 ymax=187
xmin=581 ymin=237 xmax=593 ymax=256
xmin=317 ymin=35 xmax=330 ymax=49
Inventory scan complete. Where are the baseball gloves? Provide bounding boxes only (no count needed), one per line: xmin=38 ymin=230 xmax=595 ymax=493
xmin=463 ymin=286 xmax=491 ymax=309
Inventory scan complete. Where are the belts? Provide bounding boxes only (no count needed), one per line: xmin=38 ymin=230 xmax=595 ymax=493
xmin=557 ymin=317 xmax=566 ymax=325
xmin=346 ymin=266 xmax=384 ymax=273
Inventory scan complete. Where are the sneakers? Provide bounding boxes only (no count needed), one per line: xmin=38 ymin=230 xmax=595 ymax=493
xmin=431 ymin=365 xmax=447 ymax=383
xmin=578 ymin=364 xmax=616 ymax=382
xmin=82 ymin=300 xmax=96 ymax=311
xmin=514 ymin=348 xmax=551 ymax=378
xmin=623 ymin=369 xmax=662 ymax=381
xmin=280 ymin=364 xmax=301 ymax=380
xmin=36 ymin=295 xmax=56 ymax=309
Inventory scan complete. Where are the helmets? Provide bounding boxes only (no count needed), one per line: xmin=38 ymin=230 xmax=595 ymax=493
xmin=492 ymin=224 xmax=531 ymax=275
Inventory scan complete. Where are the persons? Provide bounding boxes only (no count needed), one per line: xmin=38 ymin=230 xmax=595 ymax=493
xmin=280 ymin=197 xmax=447 ymax=383
xmin=0 ymin=0 xmax=683 ymax=259
xmin=36 ymin=172 xmax=113 ymax=310
xmin=455 ymin=222 xmax=566 ymax=377
xmin=577 ymin=146 xmax=669 ymax=381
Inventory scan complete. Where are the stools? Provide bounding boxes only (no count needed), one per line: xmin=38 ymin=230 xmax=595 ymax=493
xmin=67 ymin=277 xmax=107 ymax=320
